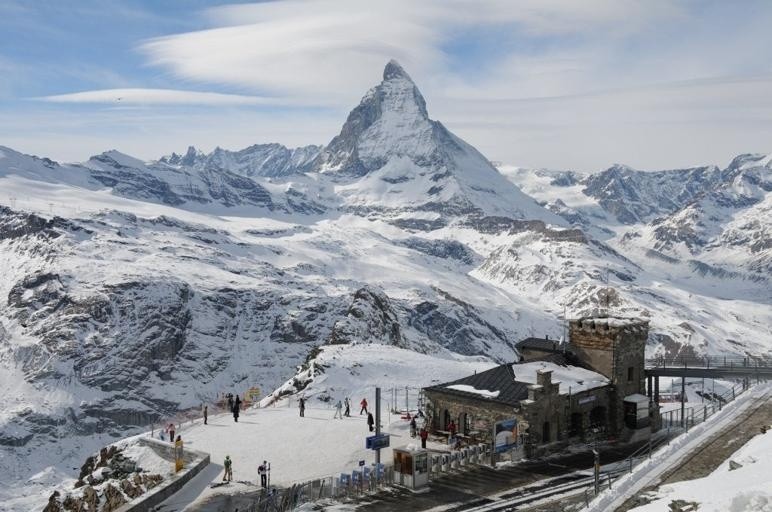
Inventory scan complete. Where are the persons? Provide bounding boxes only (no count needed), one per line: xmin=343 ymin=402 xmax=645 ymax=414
xmin=229 ymin=394 xmax=242 ymax=423
xmin=334 ymin=400 xmax=344 ymax=420
xmin=360 ymin=399 xmax=369 ymax=414
xmin=448 ymin=420 xmax=456 ymax=436
xmin=298 ymin=399 xmax=305 ymax=417
xmin=410 ymin=409 xmax=428 ymax=448
xmin=259 ymin=460 xmax=271 ymax=485
xmin=203 ymin=406 xmax=209 ymax=425
xmin=223 ymin=457 xmax=233 ymax=480
xmin=168 ymin=425 xmax=177 ymax=442
xmin=367 ymin=413 xmax=374 ymax=431
xmin=343 ymin=397 xmax=353 ymax=417
